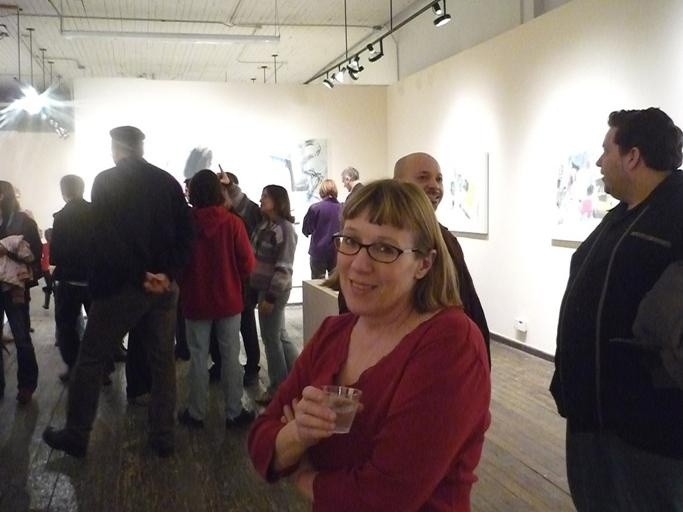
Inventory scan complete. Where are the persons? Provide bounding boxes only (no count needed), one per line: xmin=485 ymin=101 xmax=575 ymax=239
xmin=338 ymin=153 xmax=490 ymax=373
xmin=245 ymin=182 xmax=492 ymax=512
xmin=549 ymin=107 xmax=683 ymax=512
xmin=341 ymin=166 xmax=365 ymax=204
xmin=302 ymin=179 xmax=344 ymax=279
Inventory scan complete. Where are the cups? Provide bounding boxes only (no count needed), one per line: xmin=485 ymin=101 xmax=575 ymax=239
xmin=320 ymin=385 xmax=362 ymax=434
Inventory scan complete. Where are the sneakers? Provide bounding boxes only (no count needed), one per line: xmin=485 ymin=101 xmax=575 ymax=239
xmin=43 ymin=426 xmax=86 ymax=458
xmin=226 ymin=408 xmax=255 ymax=429
xmin=209 ymin=361 xmax=220 ymax=375
xmin=244 ymin=365 xmax=261 ymax=384
xmin=255 ymin=386 xmax=272 ymax=403
xmin=178 ymin=408 xmax=203 ymax=429
xmin=16 ymin=389 xmax=32 ymax=402
xmin=128 ymin=391 xmax=152 ymax=406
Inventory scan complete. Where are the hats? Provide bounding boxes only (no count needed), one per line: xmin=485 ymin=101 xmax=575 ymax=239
xmin=110 ymin=126 xmax=144 ymax=141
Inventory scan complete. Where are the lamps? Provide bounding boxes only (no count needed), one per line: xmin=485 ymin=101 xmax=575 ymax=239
xmin=322 ymin=0 xmax=452 ymax=91
xmin=0 ymin=4 xmax=71 ymax=140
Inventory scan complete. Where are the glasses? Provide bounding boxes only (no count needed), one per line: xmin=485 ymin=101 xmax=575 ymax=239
xmin=331 ymin=230 xmax=424 ymax=264
